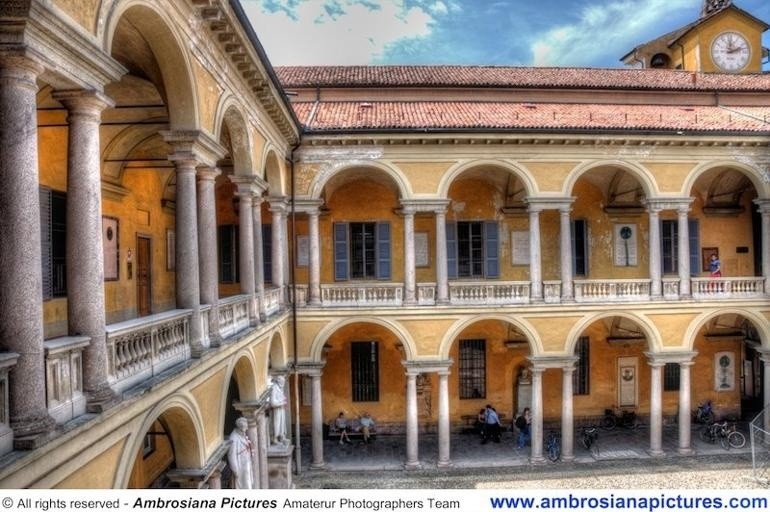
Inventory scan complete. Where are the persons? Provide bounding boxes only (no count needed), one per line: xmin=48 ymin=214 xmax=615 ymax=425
xmin=472 ymin=404 xmax=504 ymax=445
xmin=269 ymin=375 xmax=290 ymax=446
xmin=227 ymin=416 xmax=256 ymax=489
xmin=708 ymin=254 xmax=726 ymax=293
xmin=335 ymin=412 xmax=352 ymax=446
xmin=516 ymin=408 xmax=532 ymax=451
xmin=359 ymin=412 xmax=375 ymax=444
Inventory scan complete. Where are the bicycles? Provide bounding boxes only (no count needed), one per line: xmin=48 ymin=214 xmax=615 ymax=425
xmin=577 ymin=421 xmax=601 ymax=462
xmin=599 ymin=410 xmax=640 ymax=431
xmin=546 ymin=428 xmax=563 ymax=466
xmin=691 ymin=400 xmax=746 ymax=451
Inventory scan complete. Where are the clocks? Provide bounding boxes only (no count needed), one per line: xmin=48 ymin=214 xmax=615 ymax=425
xmin=710 ymin=30 xmax=753 ymax=74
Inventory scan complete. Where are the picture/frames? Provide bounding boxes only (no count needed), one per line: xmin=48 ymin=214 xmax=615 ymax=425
xmin=509 ymin=229 xmax=531 ymax=267
xmin=612 ymin=221 xmax=639 ymax=266
xmin=296 ymin=234 xmax=322 ymax=268
xmin=702 ymin=247 xmax=719 ymax=271
xmin=166 ymin=227 xmax=176 ymax=271
xmin=101 ymin=215 xmax=119 ymax=281
xmin=413 ymin=230 xmax=431 ymax=268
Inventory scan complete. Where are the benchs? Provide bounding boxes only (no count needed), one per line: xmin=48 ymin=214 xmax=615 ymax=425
xmin=463 ymin=413 xmax=516 ymax=439
xmin=326 ymin=419 xmax=377 ymax=441
